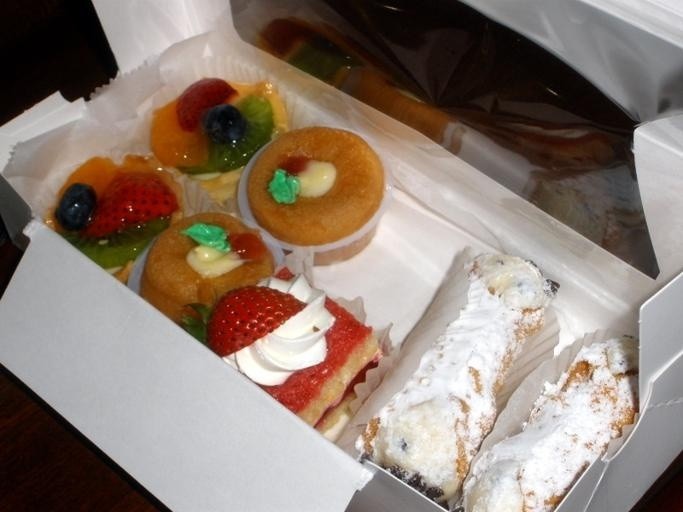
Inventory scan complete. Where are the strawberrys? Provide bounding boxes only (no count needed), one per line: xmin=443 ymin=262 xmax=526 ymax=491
xmin=208 ymin=285 xmax=308 ymax=357
xmin=79 ymin=170 xmax=181 ymax=238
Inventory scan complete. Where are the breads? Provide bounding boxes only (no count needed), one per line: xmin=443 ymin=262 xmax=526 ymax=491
xmin=457 ymin=334 xmax=639 ymax=511
xmin=336 ymin=251 xmax=554 ymax=512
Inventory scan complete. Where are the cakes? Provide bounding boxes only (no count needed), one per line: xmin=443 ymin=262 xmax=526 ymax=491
xmin=236 ymin=125 xmax=394 ymax=266
xmin=200 ymin=266 xmax=393 ymax=444
xmin=128 ymin=210 xmax=285 ymax=342
xmin=143 ymin=78 xmax=291 ymax=214
xmin=41 ymin=148 xmax=187 ymax=288
